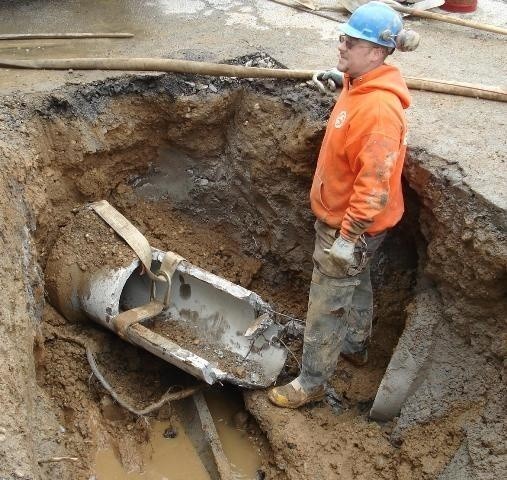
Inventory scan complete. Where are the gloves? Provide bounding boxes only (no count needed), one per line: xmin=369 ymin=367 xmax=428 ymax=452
xmin=322 ymin=68 xmax=342 ymax=86
xmin=328 ymin=237 xmax=355 ymax=266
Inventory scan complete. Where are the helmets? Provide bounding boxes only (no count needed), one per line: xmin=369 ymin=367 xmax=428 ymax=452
xmin=340 ymin=1 xmax=403 ymax=48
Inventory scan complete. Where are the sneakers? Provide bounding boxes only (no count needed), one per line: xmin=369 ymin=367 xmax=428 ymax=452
xmin=341 ymin=349 xmax=368 ymax=365
xmin=268 ymin=377 xmax=326 ymax=409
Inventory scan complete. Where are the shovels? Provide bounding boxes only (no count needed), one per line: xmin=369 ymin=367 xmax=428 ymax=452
xmin=337 ymin=0 xmax=507 ymax=35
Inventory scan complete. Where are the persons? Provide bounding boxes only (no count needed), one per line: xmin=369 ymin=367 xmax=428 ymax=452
xmin=266 ymin=1 xmax=412 ymax=408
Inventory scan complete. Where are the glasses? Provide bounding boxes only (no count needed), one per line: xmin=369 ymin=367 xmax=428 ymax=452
xmin=339 ymin=35 xmax=379 ymax=49
